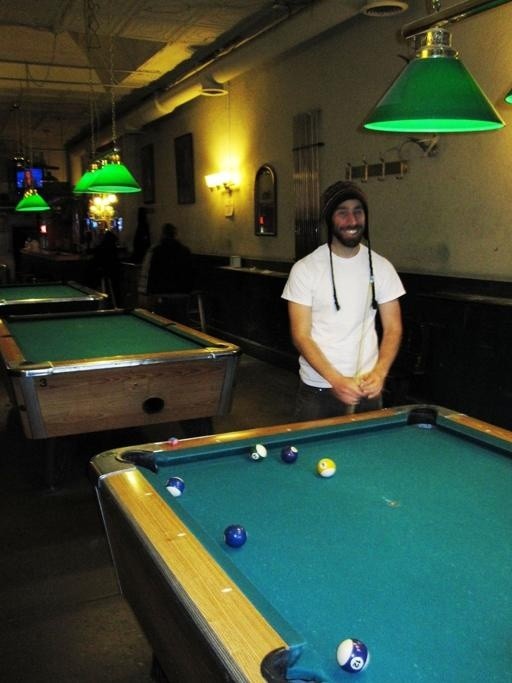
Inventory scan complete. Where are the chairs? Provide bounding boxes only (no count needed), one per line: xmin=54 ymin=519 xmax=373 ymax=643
xmin=92 ymin=246 xmax=210 ymax=335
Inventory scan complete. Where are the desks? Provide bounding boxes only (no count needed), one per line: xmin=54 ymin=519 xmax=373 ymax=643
xmin=0 ymin=276 xmax=112 ymax=317
xmin=0 ymin=300 xmax=245 ymax=503
xmin=80 ymin=396 xmax=511 ymax=683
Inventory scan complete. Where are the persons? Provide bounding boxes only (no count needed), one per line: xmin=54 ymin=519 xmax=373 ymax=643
xmin=280 ymin=180 xmax=407 ymax=423
xmin=88 ymin=231 xmax=123 ymax=281
xmin=148 ymin=222 xmax=201 ymax=296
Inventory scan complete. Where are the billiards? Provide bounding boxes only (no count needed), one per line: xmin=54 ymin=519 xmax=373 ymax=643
xmin=317 ymin=458 xmax=336 ymax=478
xmin=249 ymin=444 xmax=267 ymax=462
xmin=336 ymin=639 xmax=371 ymax=674
xmin=164 ymin=476 xmax=185 ymax=498
xmin=281 ymin=446 xmax=299 ymax=463
xmin=223 ymin=525 xmax=248 ymax=547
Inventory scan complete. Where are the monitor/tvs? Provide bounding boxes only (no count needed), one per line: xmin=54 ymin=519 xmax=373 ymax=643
xmin=16 ymin=168 xmax=44 ymax=189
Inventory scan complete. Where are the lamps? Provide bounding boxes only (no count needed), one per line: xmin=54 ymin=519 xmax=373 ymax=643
xmin=351 ymin=0 xmax=512 ymax=138
xmin=7 ymin=0 xmax=146 ymax=221
xmin=203 ymin=169 xmax=241 ymax=197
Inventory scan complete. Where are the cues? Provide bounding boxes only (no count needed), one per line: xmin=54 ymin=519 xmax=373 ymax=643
xmin=346 ymin=276 xmax=374 ymax=416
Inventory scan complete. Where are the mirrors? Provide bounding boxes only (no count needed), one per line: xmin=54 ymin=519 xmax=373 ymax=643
xmin=250 ymin=160 xmax=279 ymax=236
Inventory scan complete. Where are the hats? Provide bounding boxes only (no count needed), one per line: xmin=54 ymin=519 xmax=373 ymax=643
xmin=320 ymin=179 xmax=369 ymax=223
